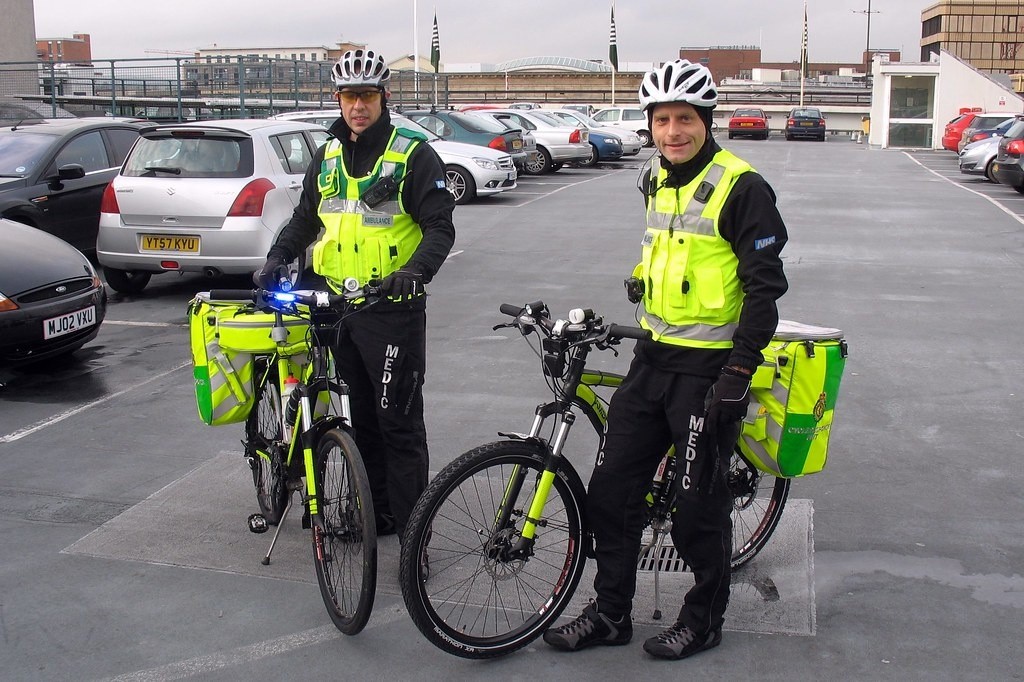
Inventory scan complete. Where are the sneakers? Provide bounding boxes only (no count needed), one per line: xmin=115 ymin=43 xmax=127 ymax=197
xmin=543 ymin=597 xmax=633 ymax=651
xmin=643 ymin=621 xmax=723 ymax=660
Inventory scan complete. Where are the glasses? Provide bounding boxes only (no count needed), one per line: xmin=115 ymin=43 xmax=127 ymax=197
xmin=337 ymin=90 xmax=384 ymax=103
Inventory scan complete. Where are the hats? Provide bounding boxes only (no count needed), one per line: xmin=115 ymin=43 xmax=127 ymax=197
xmin=330 ymin=49 xmax=391 ymax=88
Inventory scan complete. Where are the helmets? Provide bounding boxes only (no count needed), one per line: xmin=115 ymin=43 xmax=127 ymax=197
xmin=638 ymin=58 xmax=719 ymax=111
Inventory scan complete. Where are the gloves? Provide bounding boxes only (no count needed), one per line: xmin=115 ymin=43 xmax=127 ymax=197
xmin=703 ymin=366 xmax=753 ymax=435
xmin=379 ymin=269 xmax=427 ymax=307
xmin=254 ymin=254 xmax=288 ymax=292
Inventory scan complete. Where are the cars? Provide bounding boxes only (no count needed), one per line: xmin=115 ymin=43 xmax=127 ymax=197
xmin=0 ymin=102 xmax=334 ymax=300
xmin=0 ymin=217 xmax=107 ymax=370
xmin=267 ymin=108 xmax=518 ymax=206
xmin=783 ymin=107 xmax=828 ymax=142
xmin=942 ymin=112 xmax=1024 ymax=195
xmin=396 ymin=103 xmax=654 ymax=175
xmin=727 ymin=108 xmax=771 ymax=139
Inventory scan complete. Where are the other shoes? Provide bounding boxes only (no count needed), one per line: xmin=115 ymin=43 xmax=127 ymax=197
xmin=423 ymin=546 xmax=430 ymax=583
xmin=333 ymin=517 xmax=398 ymax=542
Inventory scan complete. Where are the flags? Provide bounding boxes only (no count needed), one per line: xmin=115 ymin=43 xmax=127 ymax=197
xmin=609 ymin=6 xmax=618 ymax=73
xmin=798 ymin=10 xmax=809 ymax=80
xmin=431 ymin=13 xmax=440 ymax=73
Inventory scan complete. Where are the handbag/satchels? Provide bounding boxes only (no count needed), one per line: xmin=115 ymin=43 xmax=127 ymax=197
xmin=186 ymin=291 xmax=337 ymax=426
xmin=735 ymin=318 xmax=849 ymax=480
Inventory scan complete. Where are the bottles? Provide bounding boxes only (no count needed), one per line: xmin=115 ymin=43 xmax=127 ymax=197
xmin=285 ymin=383 xmax=307 ymax=426
xmin=282 ymin=375 xmax=298 ymax=444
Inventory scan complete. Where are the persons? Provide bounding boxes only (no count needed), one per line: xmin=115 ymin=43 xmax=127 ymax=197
xmin=543 ymin=59 xmax=787 ymax=662
xmin=255 ymin=46 xmax=455 ymax=582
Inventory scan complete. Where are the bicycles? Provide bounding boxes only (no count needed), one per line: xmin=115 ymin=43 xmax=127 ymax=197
xmin=210 ymin=284 xmax=427 ymax=636
xmin=398 ymin=303 xmax=792 ymax=659
